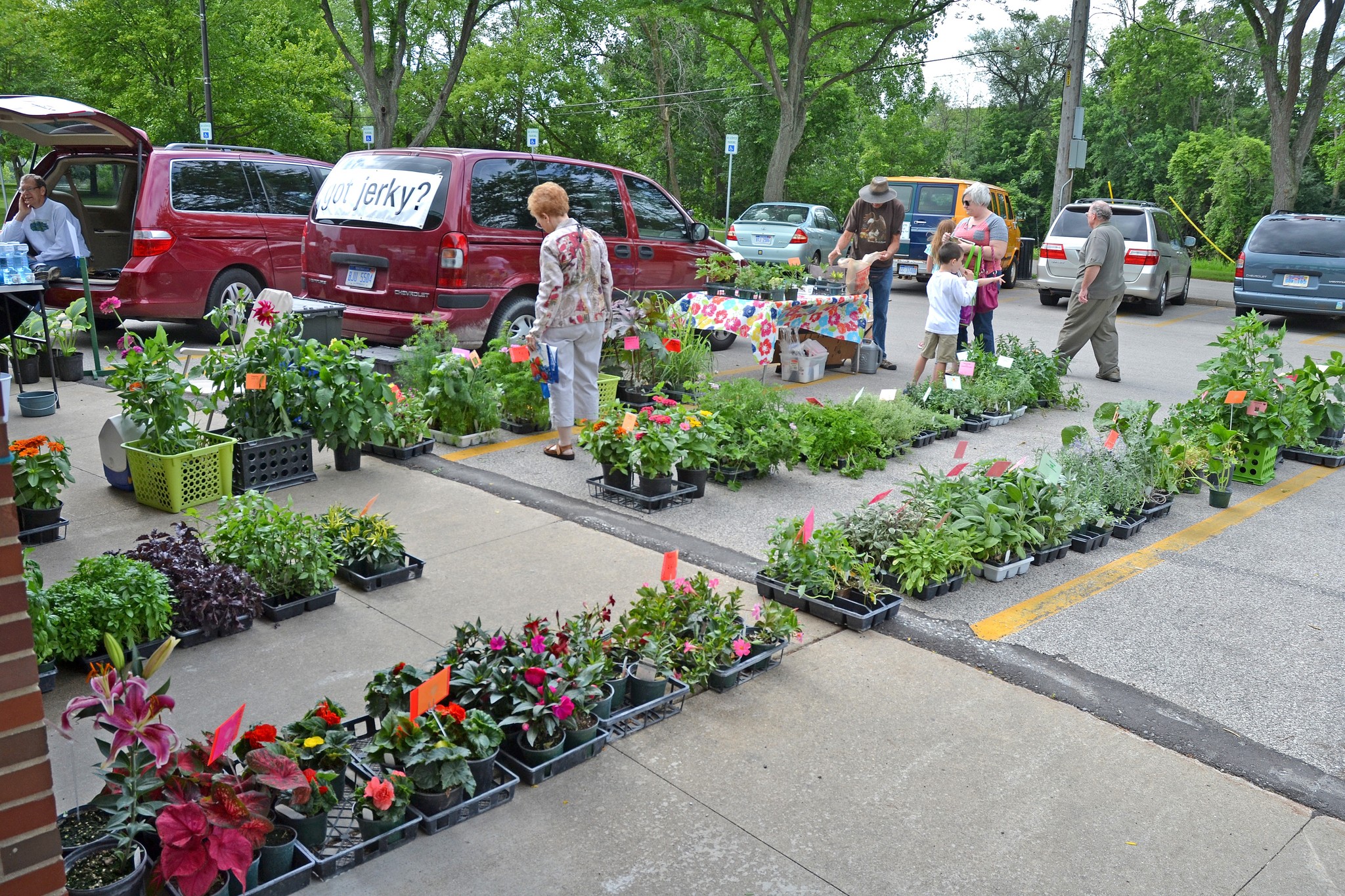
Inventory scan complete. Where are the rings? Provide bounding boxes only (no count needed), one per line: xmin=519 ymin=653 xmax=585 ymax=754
xmin=528 ymin=343 xmax=531 ymax=345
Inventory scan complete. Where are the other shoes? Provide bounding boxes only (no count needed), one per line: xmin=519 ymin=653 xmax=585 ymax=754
xmin=942 ymin=368 xmax=959 ymax=378
xmin=918 ymin=341 xmax=925 ymax=349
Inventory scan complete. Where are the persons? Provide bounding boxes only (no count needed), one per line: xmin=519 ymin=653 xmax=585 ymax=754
xmin=917 ymin=218 xmax=956 ymax=349
xmin=902 ymin=242 xmax=1005 ymax=397
xmin=828 ymin=176 xmax=905 ymax=371
xmin=525 ymin=181 xmax=613 ymax=461
xmin=0 ymin=173 xmax=92 ymax=333
xmin=1046 ymin=200 xmax=1125 ymax=382
xmin=940 ymin=182 xmax=1009 ymax=380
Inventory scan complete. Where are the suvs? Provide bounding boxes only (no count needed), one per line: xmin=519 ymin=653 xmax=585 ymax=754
xmin=1233 ymin=209 xmax=1345 ymax=330
xmin=1036 ymin=198 xmax=1196 ymax=316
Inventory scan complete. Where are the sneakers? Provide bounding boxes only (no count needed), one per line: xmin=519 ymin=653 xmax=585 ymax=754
xmin=1096 ymin=369 xmax=1121 ymax=382
xmin=879 ymin=359 xmax=897 ymax=370
xmin=903 ymin=386 xmax=915 ymax=396
xmin=31 ymin=265 xmax=62 ymax=283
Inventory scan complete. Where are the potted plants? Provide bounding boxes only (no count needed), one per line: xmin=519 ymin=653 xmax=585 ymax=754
xmin=17 ymin=253 xmax=1345 ymax=896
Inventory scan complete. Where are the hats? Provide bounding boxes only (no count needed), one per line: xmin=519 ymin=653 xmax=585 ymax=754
xmin=858 ymin=176 xmax=897 ymax=204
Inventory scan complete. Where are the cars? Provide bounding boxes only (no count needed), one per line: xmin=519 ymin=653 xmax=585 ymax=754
xmin=726 ymin=202 xmax=853 ymax=279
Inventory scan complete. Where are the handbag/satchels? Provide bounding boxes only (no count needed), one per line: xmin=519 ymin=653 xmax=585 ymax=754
xmin=837 ymin=251 xmax=883 ymax=295
xmin=975 ymin=245 xmax=999 ymax=313
xmin=958 ymin=244 xmax=982 ymax=329
xmin=527 ymin=342 xmax=559 ymax=399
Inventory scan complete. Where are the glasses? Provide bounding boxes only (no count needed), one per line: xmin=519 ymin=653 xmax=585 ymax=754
xmin=1085 ymin=212 xmax=1093 ymax=217
xmin=18 ymin=186 xmax=42 ymax=193
xmin=962 ymin=200 xmax=974 ymax=206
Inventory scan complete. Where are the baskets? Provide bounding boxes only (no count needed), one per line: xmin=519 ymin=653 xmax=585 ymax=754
xmin=120 ymin=429 xmax=238 ymax=514
xmin=574 ymin=373 xmax=622 ymax=426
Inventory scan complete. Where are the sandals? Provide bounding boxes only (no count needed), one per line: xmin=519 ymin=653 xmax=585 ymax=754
xmin=577 ymin=436 xmax=589 ymax=448
xmin=543 ymin=443 xmax=575 ymax=460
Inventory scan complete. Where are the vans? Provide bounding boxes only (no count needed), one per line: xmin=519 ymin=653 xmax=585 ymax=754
xmin=874 ymin=176 xmax=1027 ymax=289
xmin=0 ymin=92 xmax=340 ymax=347
xmin=300 ymin=147 xmax=750 ymax=352
